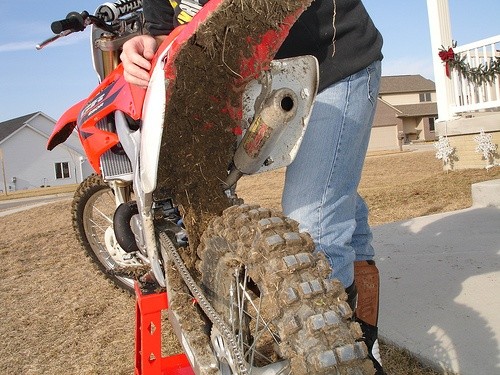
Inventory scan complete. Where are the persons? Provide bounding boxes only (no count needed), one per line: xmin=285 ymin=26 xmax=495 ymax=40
xmin=120 ymin=0 xmax=385 ymax=375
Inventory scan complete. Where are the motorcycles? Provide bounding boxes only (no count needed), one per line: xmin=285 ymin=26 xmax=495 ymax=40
xmin=36 ymin=0 xmax=377 ymax=375
